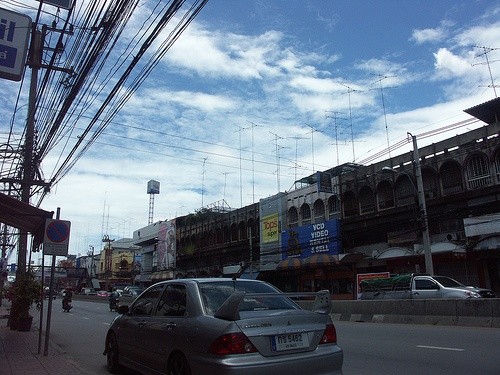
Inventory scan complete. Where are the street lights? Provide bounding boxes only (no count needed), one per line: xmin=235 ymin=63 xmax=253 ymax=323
xmin=381 ymin=166 xmax=434 ymax=276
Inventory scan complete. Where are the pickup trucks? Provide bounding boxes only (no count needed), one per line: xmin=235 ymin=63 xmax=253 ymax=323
xmin=360 ymin=272 xmax=498 ymax=298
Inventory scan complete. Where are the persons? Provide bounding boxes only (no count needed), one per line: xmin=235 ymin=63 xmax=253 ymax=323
xmin=108 ymin=287 xmax=120 ymax=305
xmin=62 ymin=287 xmax=72 ymax=309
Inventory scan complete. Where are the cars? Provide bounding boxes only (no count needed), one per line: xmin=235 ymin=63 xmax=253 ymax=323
xmin=109 ymin=290 xmax=139 ymax=314
xmin=103 ymin=278 xmax=344 ymax=375
xmin=45 ymin=285 xmax=144 ymax=300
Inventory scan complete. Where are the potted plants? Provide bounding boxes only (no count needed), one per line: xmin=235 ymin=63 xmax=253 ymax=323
xmin=6 ymin=271 xmax=47 ymax=333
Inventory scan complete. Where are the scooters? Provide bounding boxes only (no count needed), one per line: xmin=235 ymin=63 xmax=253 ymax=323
xmin=62 ymin=295 xmax=73 ymax=312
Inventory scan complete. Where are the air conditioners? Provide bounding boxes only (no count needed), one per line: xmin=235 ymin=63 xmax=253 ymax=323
xmin=445 ymin=233 xmax=458 ymax=241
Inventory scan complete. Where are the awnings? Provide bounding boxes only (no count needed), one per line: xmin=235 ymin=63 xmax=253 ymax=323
xmin=417 ymin=242 xmax=467 ymax=257
xmin=150 ymin=271 xmax=173 ymax=279
xmin=303 ymin=254 xmax=337 ymax=266
xmin=0 ymin=193 xmax=55 ymax=253
xmin=470 ymin=235 xmax=500 ymax=251
xmin=276 ymin=257 xmax=303 ymax=270
xmin=374 ymin=247 xmax=416 ymax=257
